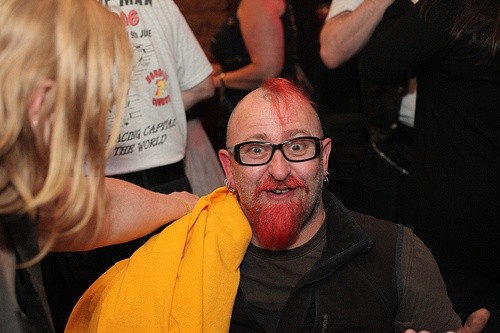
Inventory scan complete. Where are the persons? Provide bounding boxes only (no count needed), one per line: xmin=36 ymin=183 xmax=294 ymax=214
xmin=0 ymin=1 xmax=500 ymax=333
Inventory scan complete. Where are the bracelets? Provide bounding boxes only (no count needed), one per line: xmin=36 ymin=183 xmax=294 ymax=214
xmin=170 ymin=196 xmax=190 ymax=216
xmin=220 ymin=73 xmax=227 ymax=89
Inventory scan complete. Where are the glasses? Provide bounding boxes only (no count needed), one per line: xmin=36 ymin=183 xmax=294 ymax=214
xmin=225 ymin=136 xmax=326 ymax=166
